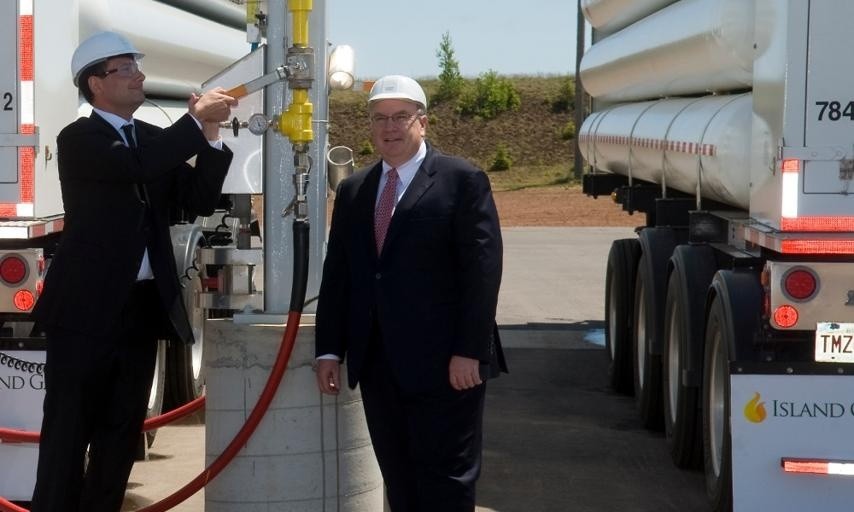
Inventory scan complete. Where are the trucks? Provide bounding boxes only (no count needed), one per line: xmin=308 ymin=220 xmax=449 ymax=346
xmin=2 ymin=1 xmax=266 ymax=503
xmin=574 ymin=1 xmax=853 ymax=511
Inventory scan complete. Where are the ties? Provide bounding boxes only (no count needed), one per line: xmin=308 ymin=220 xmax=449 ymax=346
xmin=374 ymin=169 xmax=398 ymax=257
xmin=120 ymin=125 xmax=149 ymax=208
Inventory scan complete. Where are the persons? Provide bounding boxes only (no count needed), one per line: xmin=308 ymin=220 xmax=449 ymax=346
xmin=316 ymin=76 xmax=510 ymax=512
xmin=30 ymin=33 xmax=237 ymax=506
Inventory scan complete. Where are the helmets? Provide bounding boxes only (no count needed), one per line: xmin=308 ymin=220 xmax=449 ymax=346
xmin=367 ymin=76 xmax=427 ymax=110
xmin=72 ymin=32 xmax=145 ymax=87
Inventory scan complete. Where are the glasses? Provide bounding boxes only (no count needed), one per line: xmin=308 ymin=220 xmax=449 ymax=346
xmin=100 ymin=61 xmax=138 ymax=76
xmin=370 ymin=111 xmax=421 ymax=124
